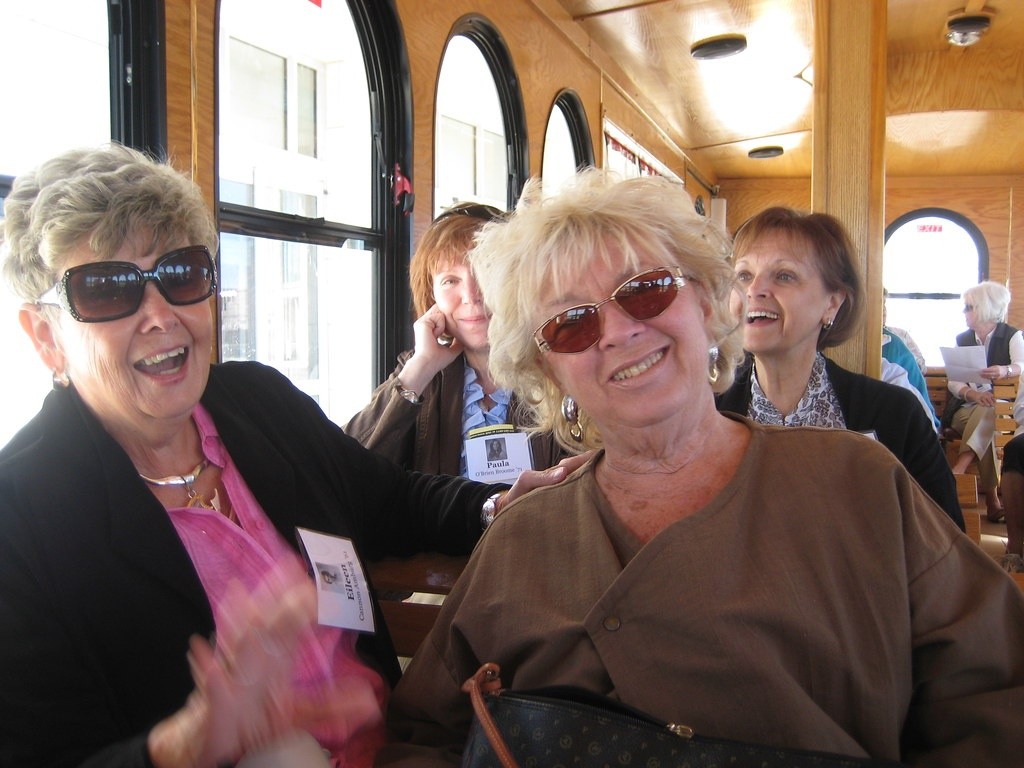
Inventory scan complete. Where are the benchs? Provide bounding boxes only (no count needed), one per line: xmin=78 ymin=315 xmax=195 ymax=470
xmin=925 ymin=367 xmax=1019 ymax=448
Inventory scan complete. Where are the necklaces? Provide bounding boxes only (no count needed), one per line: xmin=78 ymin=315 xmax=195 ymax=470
xmin=139 ymin=457 xmax=220 ymax=514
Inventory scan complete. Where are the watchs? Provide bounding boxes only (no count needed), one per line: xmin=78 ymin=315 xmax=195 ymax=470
xmin=397 ymin=385 xmax=422 ymax=404
xmin=481 ymin=489 xmax=510 ymax=535
xmin=1007 ymin=366 xmax=1012 ymax=378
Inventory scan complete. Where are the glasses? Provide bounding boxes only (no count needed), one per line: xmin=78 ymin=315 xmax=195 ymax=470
xmin=35 ymin=245 xmax=217 ymax=322
xmin=532 ymin=266 xmax=697 ymax=353
xmin=431 ymin=206 xmax=504 ymax=223
xmin=966 ymin=304 xmax=977 ymax=312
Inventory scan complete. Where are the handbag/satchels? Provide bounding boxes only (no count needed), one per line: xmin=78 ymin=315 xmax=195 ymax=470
xmin=459 ymin=662 xmax=891 ymax=768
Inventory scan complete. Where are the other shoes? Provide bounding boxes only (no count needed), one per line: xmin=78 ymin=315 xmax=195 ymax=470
xmin=987 ymin=505 xmax=1005 ymax=521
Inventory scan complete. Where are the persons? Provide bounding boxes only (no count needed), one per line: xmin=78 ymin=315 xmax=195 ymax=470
xmin=0 ymin=143 xmax=595 ymax=768
xmin=340 ymin=203 xmax=578 ymax=485
xmin=881 ymin=286 xmax=941 ymax=438
xmin=1000 ymin=367 xmax=1024 ymax=568
xmin=391 ymin=165 xmax=1024 ymax=767
xmin=940 ymin=282 xmax=1024 ymax=524
xmin=714 ymin=205 xmax=967 ymax=535
